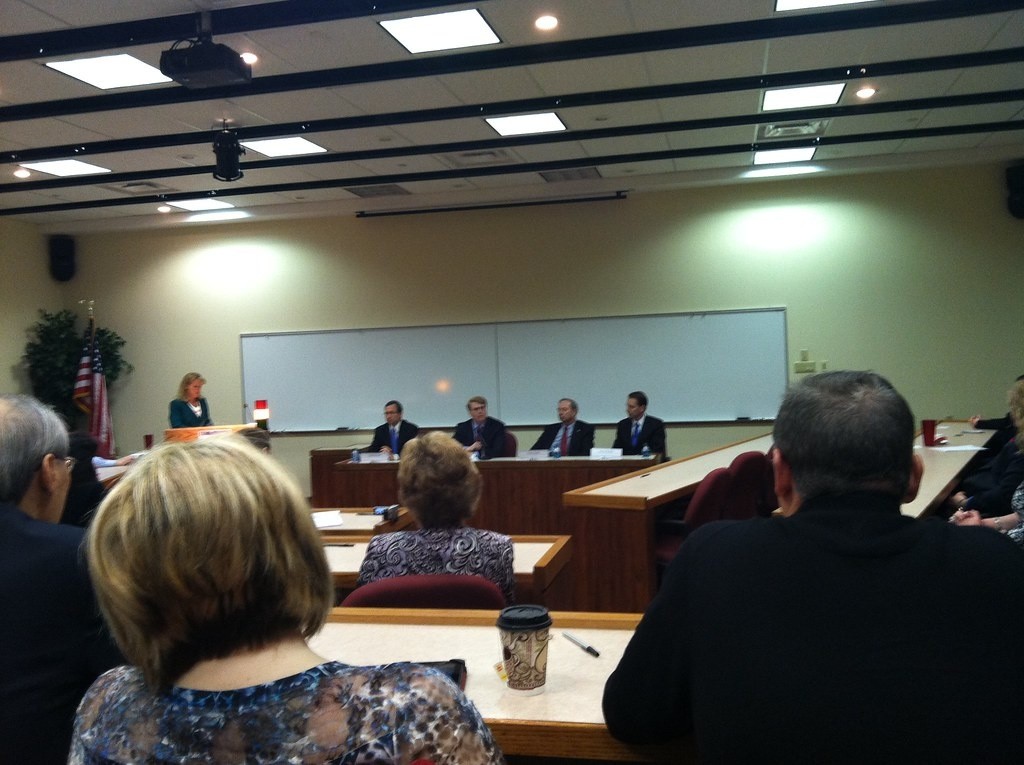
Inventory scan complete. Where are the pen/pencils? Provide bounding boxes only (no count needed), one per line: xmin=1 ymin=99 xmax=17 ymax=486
xmin=559 ymin=630 xmax=601 ymax=657
xmin=962 ymin=429 xmax=985 ymax=434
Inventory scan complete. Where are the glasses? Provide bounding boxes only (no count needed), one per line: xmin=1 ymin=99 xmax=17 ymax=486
xmin=56 ymin=455 xmax=77 ymax=473
xmin=383 ymin=411 xmax=400 ymax=415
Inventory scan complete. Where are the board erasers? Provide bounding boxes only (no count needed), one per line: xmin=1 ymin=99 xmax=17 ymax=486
xmin=737 ymin=417 xmax=750 ymax=420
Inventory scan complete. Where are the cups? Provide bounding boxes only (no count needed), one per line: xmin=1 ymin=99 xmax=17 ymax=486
xmin=922 ymin=419 xmax=935 ymax=446
xmin=495 ymin=605 xmax=553 ymax=697
xmin=143 ymin=433 xmax=154 ymax=450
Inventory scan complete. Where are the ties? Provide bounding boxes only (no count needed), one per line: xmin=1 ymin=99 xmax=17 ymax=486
xmin=632 ymin=423 xmax=640 ymax=447
xmin=477 ymin=426 xmax=486 ymax=458
xmin=560 ymin=427 xmax=568 ymax=456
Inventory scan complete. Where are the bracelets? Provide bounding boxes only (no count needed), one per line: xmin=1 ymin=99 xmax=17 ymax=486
xmin=994 ymin=516 xmax=1001 ymax=531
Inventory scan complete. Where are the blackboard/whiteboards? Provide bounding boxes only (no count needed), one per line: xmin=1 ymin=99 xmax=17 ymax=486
xmin=238 ymin=307 xmax=791 ymax=438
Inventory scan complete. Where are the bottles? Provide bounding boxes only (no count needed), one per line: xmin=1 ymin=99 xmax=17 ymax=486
xmin=551 ymin=443 xmax=561 ymax=459
xmin=642 ymin=443 xmax=649 ymax=457
xmin=350 ymin=448 xmax=360 ymax=464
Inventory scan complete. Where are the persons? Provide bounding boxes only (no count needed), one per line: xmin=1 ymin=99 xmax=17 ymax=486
xmin=92 ymin=454 xmax=132 ymax=467
xmin=928 ymin=375 xmax=1024 ymax=547
xmin=603 ymin=370 xmax=1024 ymax=765
xmin=612 ymin=392 xmax=665 ymax=463
xmin=0 ymin=393 xmax=128 ymax=765
xmin=452 ymin=395 xmax=506 ymax=459
xmin=67 ymin=435 xmax=504 ymax=765
xmin=530 ymin=398 xmax=594 ymax=457
xmin=53 ymin=396 xmax=106 ymax=530
xmin=170 ymin=372 xmax=213 ymax=427
xmin=369 ymin=400 xmax=418 ymax=460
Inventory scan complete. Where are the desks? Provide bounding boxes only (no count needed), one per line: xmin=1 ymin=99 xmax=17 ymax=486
xmin=95 ymin=444 xmax=995 ymax=763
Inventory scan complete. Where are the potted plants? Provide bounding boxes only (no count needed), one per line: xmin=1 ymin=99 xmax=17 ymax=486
xmin=21 ymin=308 xmax=132 ymax=527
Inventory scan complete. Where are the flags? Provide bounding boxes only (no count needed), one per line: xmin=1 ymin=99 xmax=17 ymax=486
xmin=73 ymin=314 xmax=115 ymax=463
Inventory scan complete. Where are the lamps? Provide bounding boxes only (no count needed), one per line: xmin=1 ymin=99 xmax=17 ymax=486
xmin=215 ymin=128 xmax=244 ymax=181
